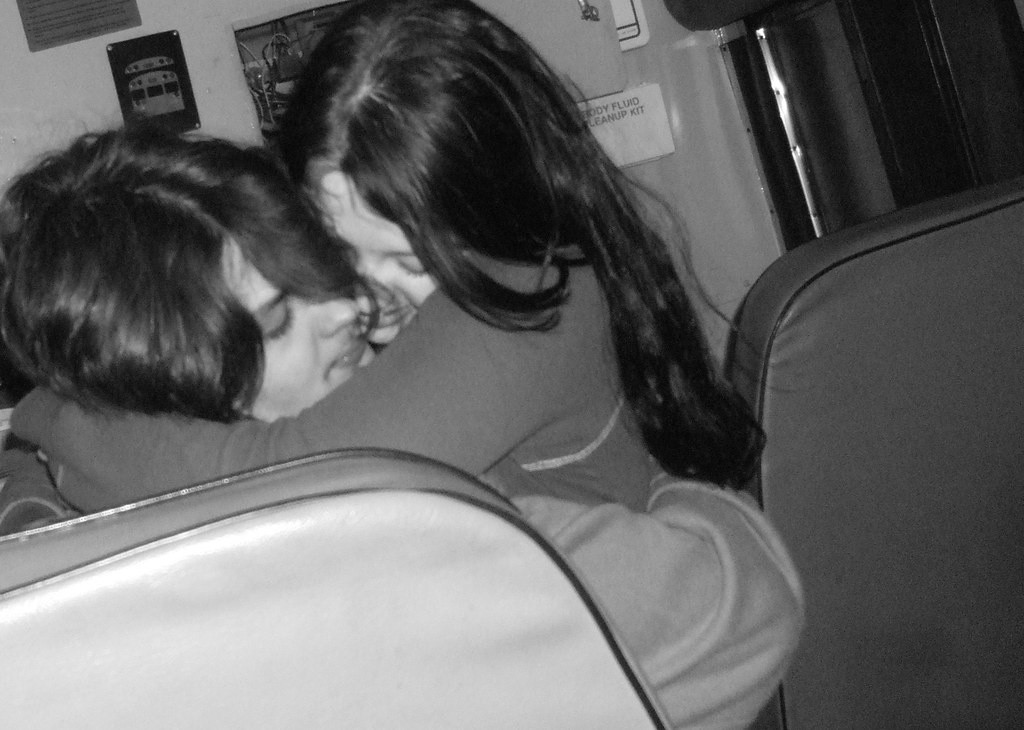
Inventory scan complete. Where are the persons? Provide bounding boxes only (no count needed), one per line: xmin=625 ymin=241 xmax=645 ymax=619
xmin=0 ymin=0 xmax=804 ymax=729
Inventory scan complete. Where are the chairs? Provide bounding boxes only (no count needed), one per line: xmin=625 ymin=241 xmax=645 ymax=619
xmin=0 ymin=426 xmax=677 ymax=730
xmin=723 ymin=175 xmax=1024 ymax=730
